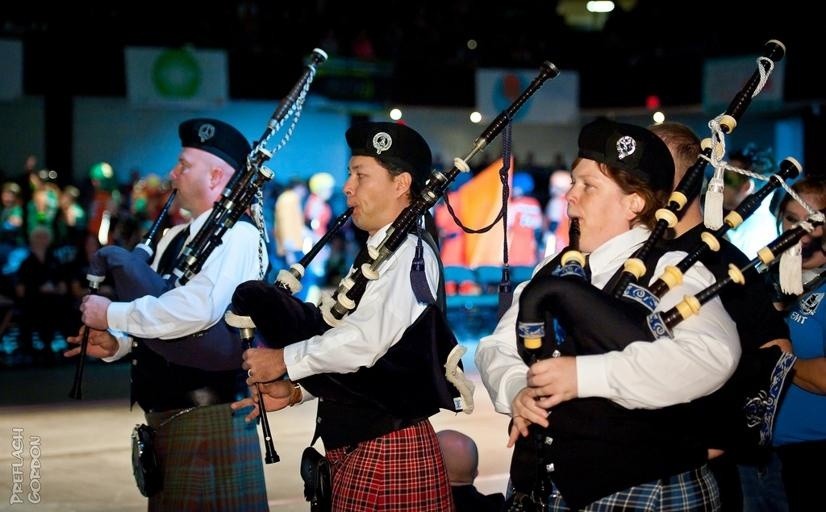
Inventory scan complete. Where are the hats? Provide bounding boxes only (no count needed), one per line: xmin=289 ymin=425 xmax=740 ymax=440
xmin=177 ymin=116 xmax=251 ymax=168
xmin=344 ymin=120 xmax=433 ymax=177
xmin=578 ymin=113 xmax=676 ymax=191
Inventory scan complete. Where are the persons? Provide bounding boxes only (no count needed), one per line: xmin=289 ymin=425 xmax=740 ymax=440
xmin=2 ymin=119 xmax=826 ymax=511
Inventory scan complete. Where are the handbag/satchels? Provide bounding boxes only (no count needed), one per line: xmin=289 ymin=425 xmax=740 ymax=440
xmin=297 ymin=448 xmax=328 ymax=512
xmin=128 ymin=423 xmax=167 ymax=498
